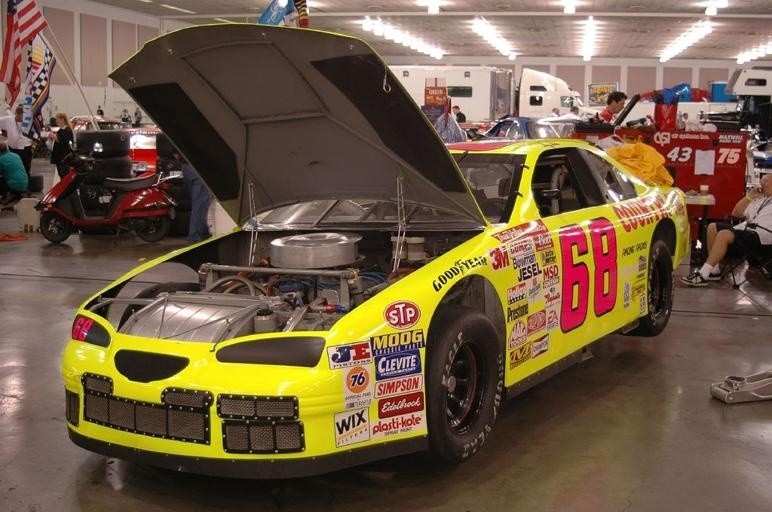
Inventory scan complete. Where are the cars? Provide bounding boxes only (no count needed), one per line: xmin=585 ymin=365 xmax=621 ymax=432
xmin=64 ymin=25 xmax=692 ymax=479
xmin=45 ymin=114 xmax=161 ymax=172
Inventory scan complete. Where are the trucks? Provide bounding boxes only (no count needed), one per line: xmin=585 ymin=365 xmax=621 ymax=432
xmin=389 ymin=63 xmax=603 ymax=124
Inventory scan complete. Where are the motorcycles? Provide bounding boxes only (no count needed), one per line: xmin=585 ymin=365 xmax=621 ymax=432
xmin=33 ymin=139 xmax=179 ymax=242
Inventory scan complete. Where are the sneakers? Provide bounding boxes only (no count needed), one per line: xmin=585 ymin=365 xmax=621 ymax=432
xmin=709 ymin=272 xmax=722 ymax=281
xmin=679 ymin=271 xmax=709 ymax=287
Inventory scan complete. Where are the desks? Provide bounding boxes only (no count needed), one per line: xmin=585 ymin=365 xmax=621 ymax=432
xmin=679 ymin=193 xmax=718 ymax=266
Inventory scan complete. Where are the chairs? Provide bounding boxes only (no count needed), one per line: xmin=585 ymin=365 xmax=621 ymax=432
xmin=531 ymin=165 xmax=562 ymax=217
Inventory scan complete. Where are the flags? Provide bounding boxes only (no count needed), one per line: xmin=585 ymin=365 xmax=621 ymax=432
xmin=1 ymin=1 xmax=49 ymax=110
xmin=20 ymin=32 xmax=57 ymax=137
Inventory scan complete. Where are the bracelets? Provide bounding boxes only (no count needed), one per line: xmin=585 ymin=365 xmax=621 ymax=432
xmin=746 ymin=196 xmax=752 ymax=202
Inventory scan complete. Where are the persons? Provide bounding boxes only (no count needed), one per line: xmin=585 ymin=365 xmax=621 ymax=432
xmin=121 ymin=109 xmax=132 ymax=123
xmin=681 ymin=112 xmax=689 ymax=131
xmin=559 ymin=106 xmax=582 ymax=120
xmin=46 ymin=113 xmax=74 ymax=181
xmin=134 ymin=107 xmax=142 ymax=127
xmin=598 ymin=90 xmax=629 ymax=124
xmin=1 ymin=103 xmax=38 ymax=198
xmin=453 ymin=105 xmax=466 ymax=124
xmin=49 ymin=118 xmax=57 ymax=127
xmin=96 ymin=105 xmax=104 ymax=117
xmin=168 ymin=149 xmax=215 ymax=247
xmin=0 ymin=140 xmax=30 ymax=211
xmin=678 ymin=173 xmax=771 ymax=287
xmin=547 ymin=108 xmax=561 ymax=118
